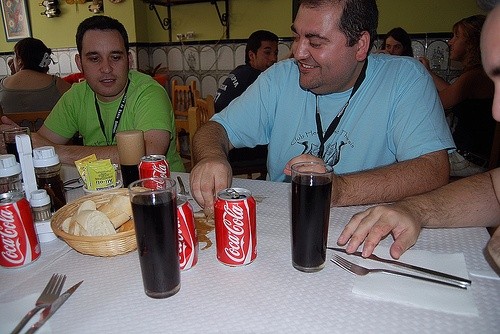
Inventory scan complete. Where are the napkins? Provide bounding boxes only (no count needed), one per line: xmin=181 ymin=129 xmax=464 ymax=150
xmin=351 ymin=247 xmax=481 ymax=317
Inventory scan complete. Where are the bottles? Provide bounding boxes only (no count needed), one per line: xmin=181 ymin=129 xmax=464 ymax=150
xmin=33 ymin=144 xmax=68 ymax=213
xmin=0 ymin=153 xmax=24 ymax=196
xmin=30 ymin=189 xmax=59 ymax=243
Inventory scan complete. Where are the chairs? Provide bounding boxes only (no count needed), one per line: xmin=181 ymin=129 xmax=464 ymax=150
xmin=171 ymin=79 xmax=261 ymax=180
xmin=0 ymin=111 xmax=50 ymax=133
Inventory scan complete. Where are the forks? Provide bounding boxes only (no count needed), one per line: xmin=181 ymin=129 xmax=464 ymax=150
xmin=10 ymin=272 xmax=68 ymax=334
xmin=177 ymin=176 xmax=192 ymax=200
xmin=329 ymin=253 xmax=467 ymax=290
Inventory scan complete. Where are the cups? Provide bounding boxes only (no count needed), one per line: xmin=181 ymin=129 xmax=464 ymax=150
xmin=115 ymin=129 xmax=147 ymax=189
xmin=2 ymin=125 xmax=33 ymax=163
xmin=291 ymin=161 xmax=335 ymax=273
xmin=81 ymin=180 xmax=123 ymax=196
xmin=127 ymin=178 xmax=181 ymax=299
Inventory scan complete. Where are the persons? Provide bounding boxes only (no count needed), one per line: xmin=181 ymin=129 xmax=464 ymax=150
xmin=0 ymin=37 xmax=72 ymax=133
xmin=0 ymin=14 xmax=187 ymax=173
xmin=416 ymin=15 xmax=497 ymax=169
xmin=337 ymin=3 xmax=500 ymax=268
xmin=380 ymin=27 xmax=414 ymax=58
xmin=189 ymin=0 xmax=457 ymax=214
xmin=213 ymin=30 xmax=279 ymax=162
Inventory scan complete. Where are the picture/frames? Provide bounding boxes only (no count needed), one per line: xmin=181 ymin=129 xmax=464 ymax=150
xmin=0 ymin=0 xmax=34 ymax=42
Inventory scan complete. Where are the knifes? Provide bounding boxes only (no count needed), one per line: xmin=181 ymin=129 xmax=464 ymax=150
xmin=24 ymin=280 xmax=84 ymax=334
xmin=326 ymin=247 xmax=472 ymax=286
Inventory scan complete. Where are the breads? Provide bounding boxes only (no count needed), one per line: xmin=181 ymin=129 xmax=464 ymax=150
xmin=62 ymin=194 xmax=135 ymax=236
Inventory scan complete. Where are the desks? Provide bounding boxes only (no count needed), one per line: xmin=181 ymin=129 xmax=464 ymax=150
xmin=0 ymin=165 xmax=500 ymax=334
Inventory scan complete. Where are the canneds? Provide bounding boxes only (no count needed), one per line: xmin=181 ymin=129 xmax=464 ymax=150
xmin=138 ymin=155 xmax=171 ymax=190
xmin=175 ymin=193 xmax=198 ymax=271
xmin=0 ymin=192 xmax=41 ymax=269
xmin=214 ymin=187 xmax=256 ymax=267
